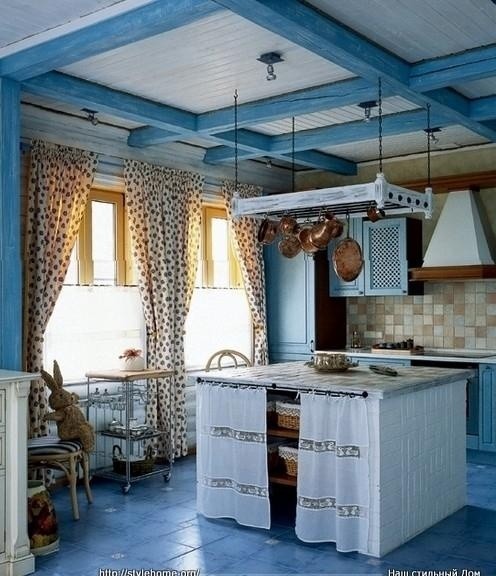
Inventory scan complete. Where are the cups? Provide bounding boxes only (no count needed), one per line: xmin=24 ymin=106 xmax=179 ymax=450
xmin=312 ymin=353 xmax=346 ymax=366
xmin=109 ymin=417 xmax=149 ymax=437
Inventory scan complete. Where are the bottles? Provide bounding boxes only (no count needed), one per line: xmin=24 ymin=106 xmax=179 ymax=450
xmin=378 ymin=338 xmax=414 ymax=350
xmin=351 ymin=331 xmax=362 ymax=347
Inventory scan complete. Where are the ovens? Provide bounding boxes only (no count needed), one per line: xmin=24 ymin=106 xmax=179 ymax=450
xmin=410 ymin=359 xmax=480 ymax=437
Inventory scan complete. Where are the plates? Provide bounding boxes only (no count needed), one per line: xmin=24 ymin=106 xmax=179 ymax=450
xmin=304 ymin=361 xmax=359 ymax=372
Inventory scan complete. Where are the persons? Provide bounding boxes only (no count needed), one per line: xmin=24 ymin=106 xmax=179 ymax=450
xmin=40 ymin=359 xmax=95 ymax=452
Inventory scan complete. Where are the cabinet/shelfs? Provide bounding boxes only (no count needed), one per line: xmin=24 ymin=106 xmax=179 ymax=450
xmin=328 ymin=216 xmax=371 ymax=298
xmin=263 ymin=222 xmax=346 ymax=355
xmin=267 ymin=424 xmax=299 ymax=487
xmin=363 ymin=217 xmax=424 ymax=297
xmin=479 ymin=364 xmax=496 ymax=452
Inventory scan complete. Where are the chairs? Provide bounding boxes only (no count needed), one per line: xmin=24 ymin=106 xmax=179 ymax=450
xmin=206 ymin=350 xmax=251 ymax=372
xmin=27 ymin=435 xmax=94 ymax=521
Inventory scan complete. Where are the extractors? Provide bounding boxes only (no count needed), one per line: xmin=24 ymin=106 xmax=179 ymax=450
xmin=408 ymin=189 xmax=496 ymax=282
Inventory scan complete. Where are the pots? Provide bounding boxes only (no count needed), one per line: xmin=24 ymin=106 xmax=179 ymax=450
xmin=258 ymin=206 xmax=385 ymax=282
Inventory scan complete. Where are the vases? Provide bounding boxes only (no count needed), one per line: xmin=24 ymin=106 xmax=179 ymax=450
xmin=28 ymin=480 xmax=58 ymax=549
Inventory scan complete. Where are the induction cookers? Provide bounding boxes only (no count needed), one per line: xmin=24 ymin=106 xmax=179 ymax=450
xmin=414 ymin=350 xmax=496 ymax=359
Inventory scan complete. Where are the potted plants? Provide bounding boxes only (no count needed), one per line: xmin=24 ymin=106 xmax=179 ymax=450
xmin=118 ymin=348 xmax=145 ymax=371
